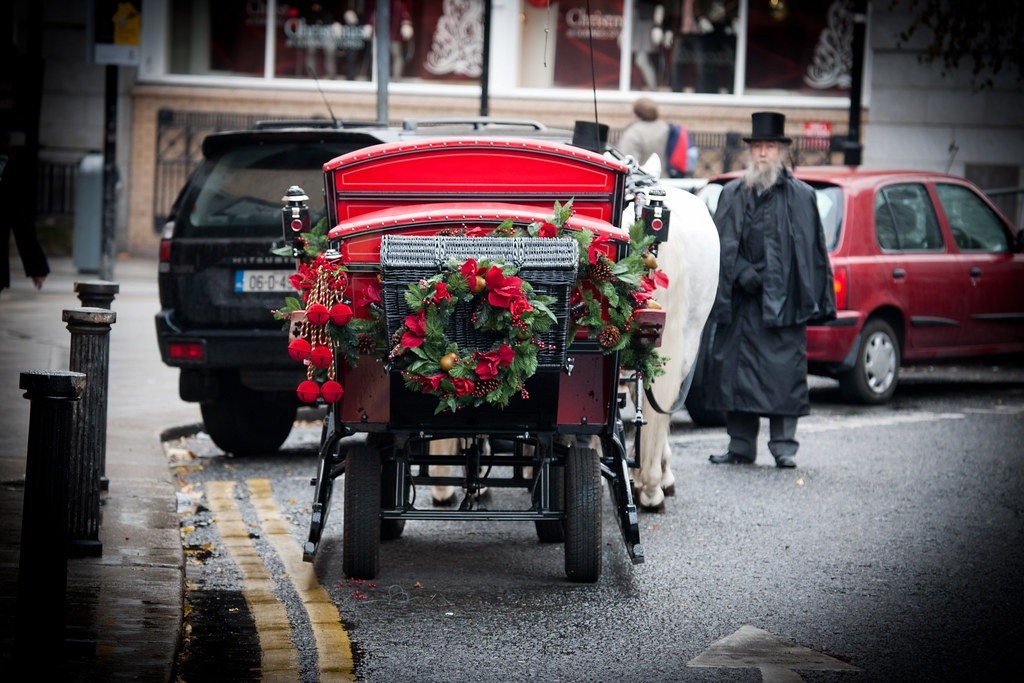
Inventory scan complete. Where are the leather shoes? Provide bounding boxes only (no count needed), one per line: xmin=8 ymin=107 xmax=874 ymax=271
xmin=709 ymin=450 xmax=754 ymax=464
xmin=776 ymin=457 xmax=797 ymax=469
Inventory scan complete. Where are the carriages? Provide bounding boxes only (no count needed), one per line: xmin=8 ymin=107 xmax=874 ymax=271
xmin=284 ymin=121 xmax=722 ymax=587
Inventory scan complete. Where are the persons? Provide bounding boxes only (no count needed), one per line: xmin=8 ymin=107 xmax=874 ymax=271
xmin=709 ymin=112 xmax=837 ymax=468
xmin=0 ymin=83 xmax=50 ymax=294
xmin=617 ymin=97 xmax=698 ymax=179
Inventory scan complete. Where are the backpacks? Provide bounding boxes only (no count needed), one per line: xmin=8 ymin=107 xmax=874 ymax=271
xmin=663 ymin=120 xmax=702 ymax=177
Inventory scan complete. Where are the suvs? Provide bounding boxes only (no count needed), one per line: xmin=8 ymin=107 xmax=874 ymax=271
xmin=154 ymin=118 xmax=572 ymax=462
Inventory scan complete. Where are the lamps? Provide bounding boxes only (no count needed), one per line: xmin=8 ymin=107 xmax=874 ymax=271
xmin=283 ymin=186 xmax=313 ymax=268
xmin=643 ymin=191 xmax=671 ymax=280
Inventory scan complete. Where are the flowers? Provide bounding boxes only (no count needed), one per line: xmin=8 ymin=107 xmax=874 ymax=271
xmin=436 ymin=199 xmax=668 ymax=356
xmin=390 ymin=258 xmax=559 ymax=415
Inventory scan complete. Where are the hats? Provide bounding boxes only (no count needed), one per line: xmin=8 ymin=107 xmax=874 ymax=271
xmin=742 ymin=111 xmax=793 ymax=146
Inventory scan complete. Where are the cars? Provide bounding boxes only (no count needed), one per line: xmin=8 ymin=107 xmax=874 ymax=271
xmin=677 ymin=165 xmax=1024 ymax=405
